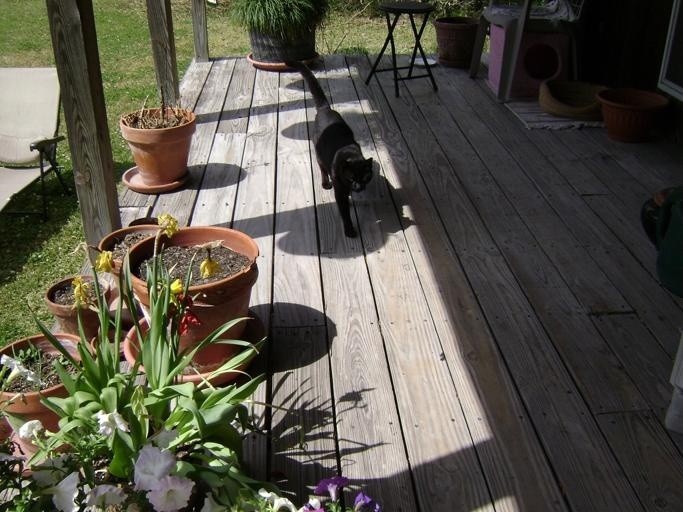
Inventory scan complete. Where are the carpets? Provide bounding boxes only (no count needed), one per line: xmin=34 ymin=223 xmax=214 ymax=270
xmin=504 ymin=101 xmax=605 ymax=131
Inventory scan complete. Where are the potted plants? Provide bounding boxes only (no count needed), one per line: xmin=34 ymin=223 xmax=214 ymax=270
xmin=45 ymin=274 xmax=111 ymax=347
xmin=119 ymin=85 xmax=197 ymax=194
xmin=124 ymin=226 xmax=265 ymax=386
xmin=1 ymin=334 xmax=93 ymax=458
xmin=230 ymin=0 xmax=330 ymax=71
xmin=97 ymin=226 xmax=169 ymax=314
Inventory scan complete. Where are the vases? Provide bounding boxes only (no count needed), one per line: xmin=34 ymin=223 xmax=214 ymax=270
xmin=434 ymin=16 xmax=480 ymax=68
xmin=595 ymin=88 xmax=670 ymax=143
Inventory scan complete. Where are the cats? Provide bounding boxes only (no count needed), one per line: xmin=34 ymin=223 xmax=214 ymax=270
xmin=284 ymin=59 xmax=373 ymax=238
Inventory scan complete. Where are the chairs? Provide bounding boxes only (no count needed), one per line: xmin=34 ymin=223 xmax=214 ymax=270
xmin=0 ymin=68 xmax=72 ymax=222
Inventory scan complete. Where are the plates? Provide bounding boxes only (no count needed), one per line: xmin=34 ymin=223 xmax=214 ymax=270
xmin=407 ymin=58 xmax=437 ymax=65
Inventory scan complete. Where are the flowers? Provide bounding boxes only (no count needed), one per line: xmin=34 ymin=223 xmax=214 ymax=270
xmin=298 ymin=476 xmax=380 ymax=512
xmin=1 ymin=213 xmax=297 ymax=512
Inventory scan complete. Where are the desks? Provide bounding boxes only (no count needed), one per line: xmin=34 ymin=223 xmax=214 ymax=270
xmin=365 ymin=2 xmax=438 ymax=97
